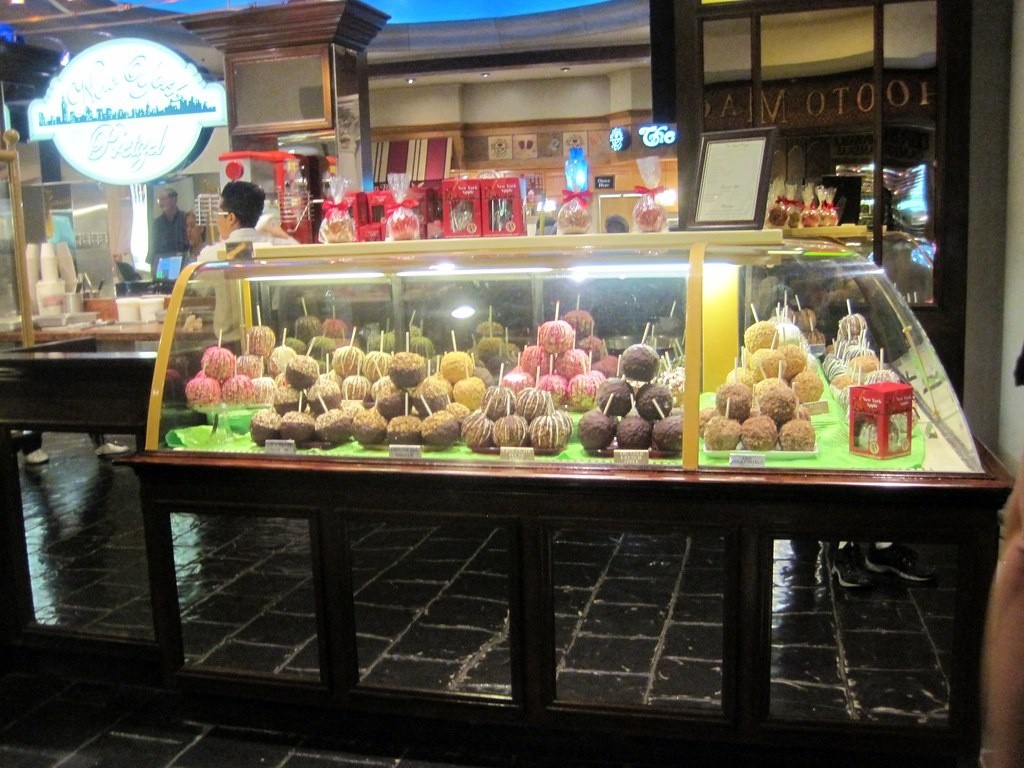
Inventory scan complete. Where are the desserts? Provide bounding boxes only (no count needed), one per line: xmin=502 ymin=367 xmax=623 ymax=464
xmin=321 ymin=199 xmax=839 ymax=241
xmin=185 ymin=311 xmax=919 ymax=451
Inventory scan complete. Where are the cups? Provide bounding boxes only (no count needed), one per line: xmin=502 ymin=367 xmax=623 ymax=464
xmin=116 ymin=297 xmax=142 ymax=323
xmin=65 ymin=292 xmax=84 ymax=313
xmin=25 ymin=240 xmax=74 ymax=308
xmin=138 ymin=297 xmax=165 ymax=324
xmin=83 ymin=290 xmax=100 ymax=299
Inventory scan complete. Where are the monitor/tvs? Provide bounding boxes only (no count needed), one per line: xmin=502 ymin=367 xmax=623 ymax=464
xmin=153 ymin=252 xmax=186 ymax=285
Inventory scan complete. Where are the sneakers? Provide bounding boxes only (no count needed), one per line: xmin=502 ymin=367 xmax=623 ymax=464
xmin=95 ymin=442 xmax=130 ymax=458
xmin=25 ymin=449 xmax=48 ymax=464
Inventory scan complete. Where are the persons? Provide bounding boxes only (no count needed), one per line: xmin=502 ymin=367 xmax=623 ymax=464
xmin=874 ymin=173 xmax=917 ymax=241
xmin=196 ymin=183 xmax=302 ymax=356
xmin=145 ymin=189 xmax=187 ymax=282
xmin=182 ymin=210 xmax=213 ymax=270
xmin=13 ymin=234 xmax=131 ymax=463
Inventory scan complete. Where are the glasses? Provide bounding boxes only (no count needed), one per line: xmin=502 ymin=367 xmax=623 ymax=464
xmin=212 ymin=208 xmax=238 ymax=220
xmin=155 ymin=196 xmax=174 ymax=204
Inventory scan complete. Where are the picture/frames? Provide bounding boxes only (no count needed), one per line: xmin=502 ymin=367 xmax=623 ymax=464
xmin=685 ymin=125 xmax=779 ymax=230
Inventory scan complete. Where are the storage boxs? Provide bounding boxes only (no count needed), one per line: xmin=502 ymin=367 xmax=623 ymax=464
xmin=848 ymin=382 xmax=916 ymax=460
xmin=481 ymin=176 xmax=546 ymax=239
xmin=319 ymin=188 xmax=434 ymax=243
xmin=442 ymin=179 xmax=483 ymax=239
